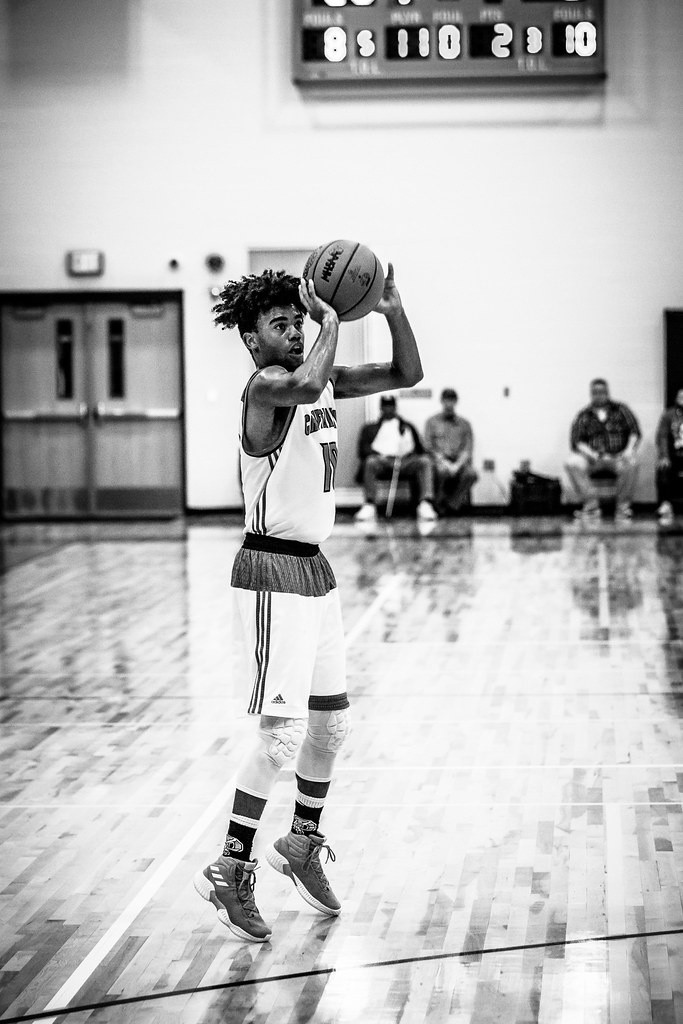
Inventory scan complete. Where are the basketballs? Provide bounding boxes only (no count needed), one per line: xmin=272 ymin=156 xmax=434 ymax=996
xmin=300 ymin=239 xmax=385 ymax=324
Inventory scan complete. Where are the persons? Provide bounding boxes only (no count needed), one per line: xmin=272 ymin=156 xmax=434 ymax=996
xmin=352 ymin=394 xmax=437 ymax=523
xmin=655 ymin=387 xmax=683 ymax=518
xmin=197 ymin=260 xmax=423 ymax=947
xmin=425 ymin=387 xmax=478 ymax=518
xmin=564 ymin=378 xmax=642 ymax=520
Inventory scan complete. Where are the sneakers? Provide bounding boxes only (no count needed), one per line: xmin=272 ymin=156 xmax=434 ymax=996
xmin=354 ymin=503 xmax=376 ymax=520
xmin=264 ymin=831 xmax=341 ymax=916
xmin=418 ymin=502 xmax=437 ymax=519
xmin=193 ymin=855 xmax=273 ymax=942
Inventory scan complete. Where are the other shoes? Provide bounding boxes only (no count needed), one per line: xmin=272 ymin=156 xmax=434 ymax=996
xmin=658 ymin=502 xmax=673 ymax=526
xmin=575 ymin=508 xmax=634 ymax=521
